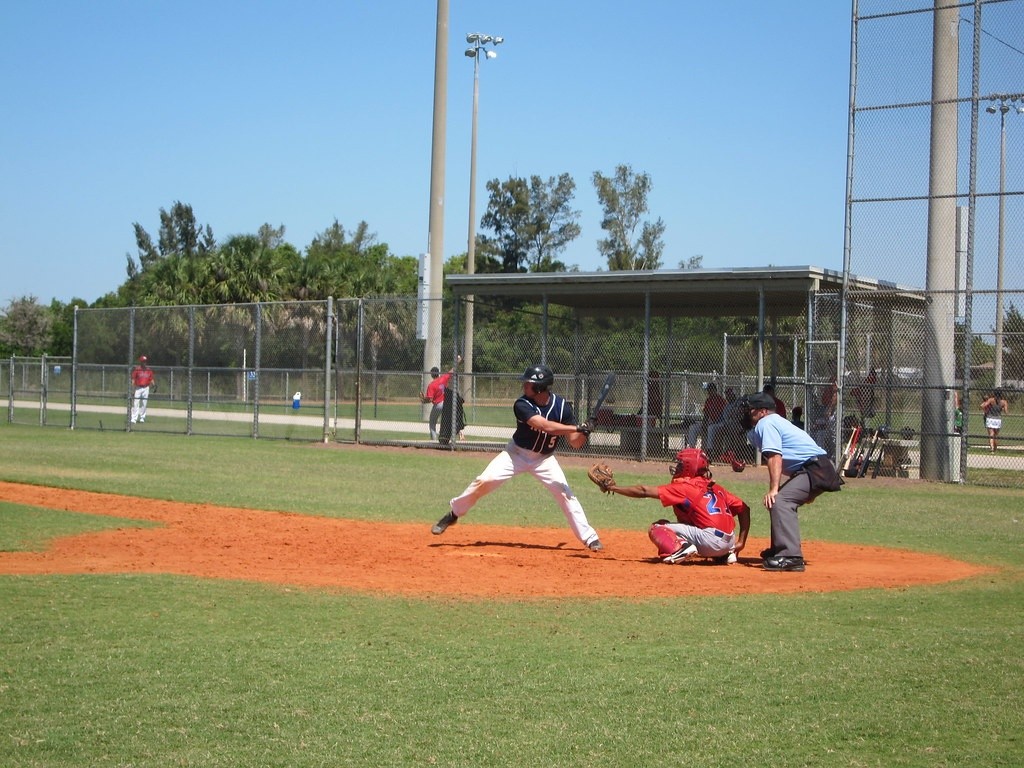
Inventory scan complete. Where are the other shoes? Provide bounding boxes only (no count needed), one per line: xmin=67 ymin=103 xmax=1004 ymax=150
xmin=589 ymin=540 xmax=603 ymax=550
xmin=990 ymin=449 xmax=1000 ymax=455
xmin=760 ymin=548 xmax=805 ymax=571
xmin=131 ymin=418 xmax=146 ymax=424
xmin=431 ymin=511 xmax=458 ymax=534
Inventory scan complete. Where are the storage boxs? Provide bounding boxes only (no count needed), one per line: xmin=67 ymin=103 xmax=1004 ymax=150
xmin=592 ymin=405 xmax=613 ymax=427
xmin=634 ymin=415 xmax=656 ymax=427
xmin=884 ymin=445 xmax=908 ymax=465
xmin=614 ymin=412 xmax=632 ymax=428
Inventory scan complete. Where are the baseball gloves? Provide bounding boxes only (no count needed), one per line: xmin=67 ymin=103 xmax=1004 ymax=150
xmin=150 ymin=385 xmax=158 ymax=393
xmin=587 ymin=460 xmax=616 ymax=497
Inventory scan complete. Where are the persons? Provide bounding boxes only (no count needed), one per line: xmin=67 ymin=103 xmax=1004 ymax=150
xmin=688 ymin=384 xmax=744 ymax=452
xmin=821 ymin=358 xmax=846 ymax=457
xmin=762 ymin=384 xmax=787 ymax=420
xmin=131 ymin=355 xmax=158 ymax=424
xmin=791 ymin=406 xmax=805 ymax=430
xmin=419 ymin=355 xmax=464 ymax=440
xmin=954 ymin=390 xmax=962 ymax=431
xmin=738 ymin=392 xmax=844 ymax=572
xmin=587 ymin=448 xmax=750 ymax=565
xmin=980 ymin=387 xmax=1009 ymax=453
xmin=431 ymin=366 xmax=603 ymax=551
xmin=636 ymin=371 xmax=662 ymax=416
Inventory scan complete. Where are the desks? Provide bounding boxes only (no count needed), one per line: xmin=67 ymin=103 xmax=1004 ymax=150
xmin=590 ymin=427 xmax=693 ymax=454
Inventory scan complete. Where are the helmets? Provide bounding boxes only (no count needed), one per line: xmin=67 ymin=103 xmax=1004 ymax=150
xmin=523 ymin=365 xmax=554 ymax=393
xmin=669 ymin=448 xmax=712 ymax=479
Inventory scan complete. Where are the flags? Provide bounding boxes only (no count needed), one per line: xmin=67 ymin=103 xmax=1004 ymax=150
xmin=853 ymin=370 xmax=877 ymax=417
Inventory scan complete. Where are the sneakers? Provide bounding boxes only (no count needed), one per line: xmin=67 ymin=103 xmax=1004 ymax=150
xmin=663 ymin=543 xmax=698 ymax=564
xmin=713 ymin=553 xmax=737 ymax=564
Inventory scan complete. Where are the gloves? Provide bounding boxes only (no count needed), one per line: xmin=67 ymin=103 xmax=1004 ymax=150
xmin=576 ymin=422 xmax=594 ymax=433
xmin=419 ymin=391 xmax=424 ymax=399
xmin=151 ymin=385 xmax=157 ymax=393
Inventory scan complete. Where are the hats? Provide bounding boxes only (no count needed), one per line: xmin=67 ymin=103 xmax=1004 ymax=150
xmin=430 ymin=367 xmax=439 ymax=373
xmin=140 ymin=356 xmax=148 ymax=362
xmin=748 ymin=392 xmax=776 ymax=412
xmin=994 ymin=387 xmax=1002 ymax=396
xmin=702 ymin=383 xmax=716 ymax=391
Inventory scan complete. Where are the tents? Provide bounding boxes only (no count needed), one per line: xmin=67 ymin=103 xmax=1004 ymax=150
xmin=860 ymin=365 xmax=923 ymax=379
xmin=960 ymin=361 xmax=1006 ymax=370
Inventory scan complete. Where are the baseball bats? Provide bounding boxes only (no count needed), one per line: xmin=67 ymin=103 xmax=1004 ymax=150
xmin=835 ymin=425 xmax=887 ymax=479
xmin=583 ymin=372 xmax=616 ymax=437
